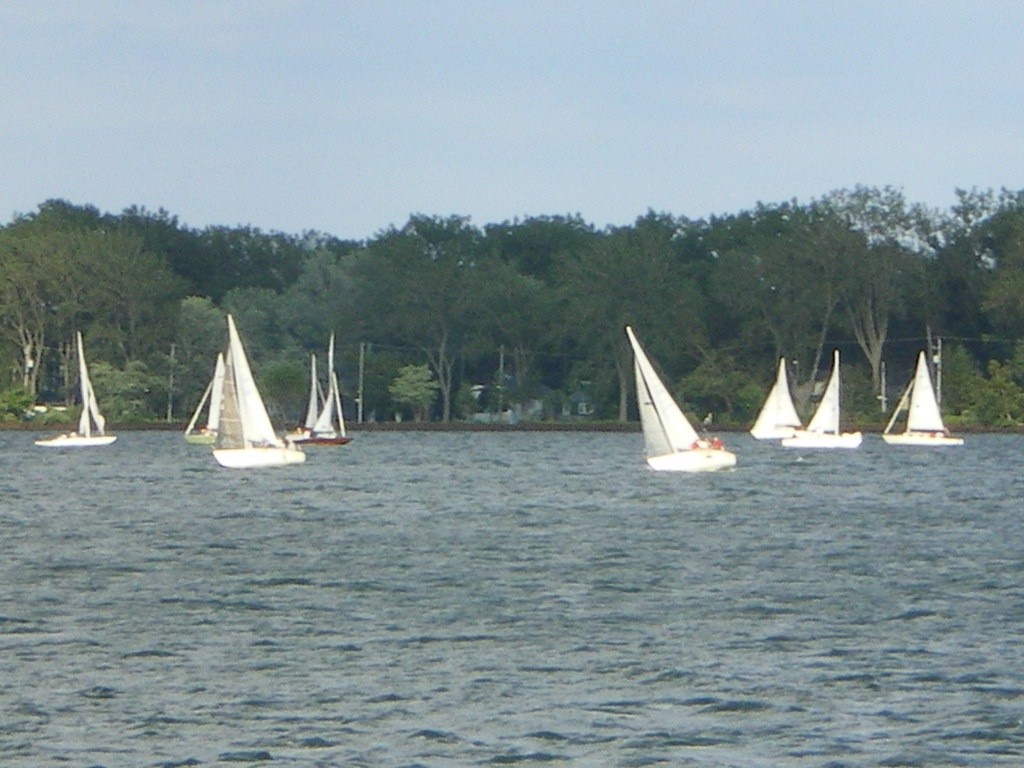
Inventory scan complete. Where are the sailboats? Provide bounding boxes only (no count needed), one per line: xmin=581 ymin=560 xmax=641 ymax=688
xmin=781 ymin=350 xmax=864 ymax=450
xmin=879 ymin=350 xmax=965 ymax=448
xmin=183 ymin=379 xmax=217 ymax=446
xmin=624 ymin=324 xmax=738 ymax=473
xmin=33 ymin=330 xmax=118 ymax=447
xmin=748 ymin=355 xmax=807 ymax=440
xmin=207 ymin=311 xmax=308 ymax=468
xmin=282 ymin=331 xmax=355 ymax=446
